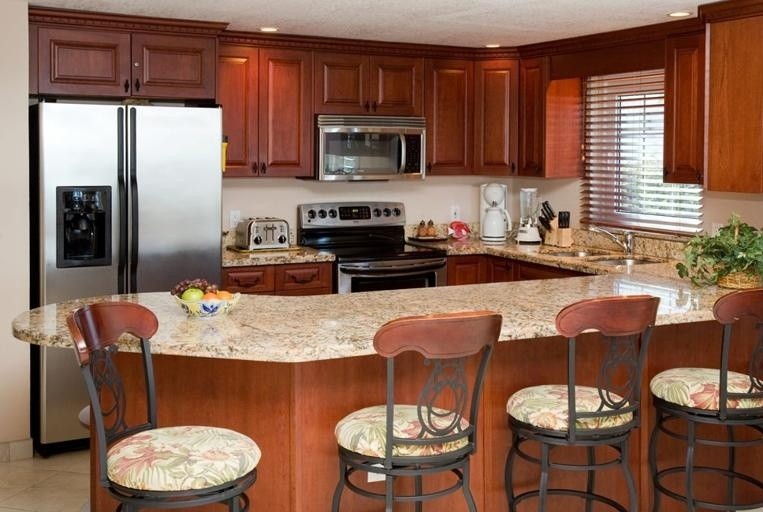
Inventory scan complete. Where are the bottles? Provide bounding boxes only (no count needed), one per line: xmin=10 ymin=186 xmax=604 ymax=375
xmin=427 ymin=219 xmax=436 ymax=237
xmin=418 ymin=220 xmax=427 ymax=237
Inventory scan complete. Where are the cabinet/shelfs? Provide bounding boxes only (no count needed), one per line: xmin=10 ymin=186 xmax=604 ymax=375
xmin=425 ymin=44 xmax=474 ymax=175
xmin=488 ymin=255 xmax=516 ymax=283
xmin=474 ymin=46 xmax=584 ymax=180
xmin=663 ymin=17 xmax=763 ymax=194
xmin=314 ymin=36 xmax=425 ymax=115
xmin=447 ymin=255 xmax=488 ymax=286
xmin=516 ymin=262 xmax=595 ymax=281
xmin=217 ymin=30 xmax=314 ymax=178
xmin=29 ymin=5 xmax=229 ymax=100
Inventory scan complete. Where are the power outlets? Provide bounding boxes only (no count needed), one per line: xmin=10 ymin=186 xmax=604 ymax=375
xmin=229 ymin=209 xmax=240 ymax=228
xmin=450 ymin=205 xmax=460 ymax=222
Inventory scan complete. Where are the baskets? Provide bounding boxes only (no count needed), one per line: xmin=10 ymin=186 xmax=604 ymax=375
xmin=713 ymin=220 xmax=762 ymax=289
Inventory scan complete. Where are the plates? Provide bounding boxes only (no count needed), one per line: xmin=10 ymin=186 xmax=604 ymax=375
xmin=407 ymin=236 xmax=448 ymax=243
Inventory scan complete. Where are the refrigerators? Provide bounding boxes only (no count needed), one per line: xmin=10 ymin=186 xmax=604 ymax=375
xmin=31 ymin=102 xmax=224 ymax=458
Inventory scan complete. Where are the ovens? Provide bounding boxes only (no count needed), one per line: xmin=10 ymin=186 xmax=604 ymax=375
xmin=333 ymin=258 xmax=447 ymax=294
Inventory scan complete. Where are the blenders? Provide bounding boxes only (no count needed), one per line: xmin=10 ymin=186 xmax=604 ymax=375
xmin=515 ymin=187 xmax=542 ymax=243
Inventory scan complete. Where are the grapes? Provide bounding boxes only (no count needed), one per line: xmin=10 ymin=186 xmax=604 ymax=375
xmin=170 ymin=278 xmax=212 ymax=296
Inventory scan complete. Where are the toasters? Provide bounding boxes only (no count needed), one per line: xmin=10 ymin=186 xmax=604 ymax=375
xmin=235 ymin=216 xmax=291 ymax=249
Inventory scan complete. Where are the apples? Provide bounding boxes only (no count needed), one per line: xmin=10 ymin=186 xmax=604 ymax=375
xmin=182 ymin=286 xmax=204 ymax=300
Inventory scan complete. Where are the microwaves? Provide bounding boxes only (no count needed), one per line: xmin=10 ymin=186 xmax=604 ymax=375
xmin=295 ymin=115 xmax=426 ymax=183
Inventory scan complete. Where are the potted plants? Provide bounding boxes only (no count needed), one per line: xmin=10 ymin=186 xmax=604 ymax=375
xmin=675 ymin=209 xmax=763 ymax=291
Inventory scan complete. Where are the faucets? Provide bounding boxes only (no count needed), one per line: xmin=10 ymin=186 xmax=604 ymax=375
xmin=587 ymin=224 xmax=635 ymax=255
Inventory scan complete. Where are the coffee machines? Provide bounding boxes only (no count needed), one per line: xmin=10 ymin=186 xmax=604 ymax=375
xmin=479 ymin=182 xmax=512 ymax=242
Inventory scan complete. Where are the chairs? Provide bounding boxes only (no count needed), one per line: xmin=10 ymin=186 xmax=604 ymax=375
xmin=332 ymin=310 xmax=503 ymax=512
xmin=65 ymin=301 xmax=261 ymax=512
xmin=648 ymin=287 xmax=763 ymax=512
xmin=504 ymin=295 xmax=660 ymax=512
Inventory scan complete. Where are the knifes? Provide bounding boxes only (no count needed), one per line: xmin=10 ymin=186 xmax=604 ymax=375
xmin=538 ymin=200 xmax=570 ymax=232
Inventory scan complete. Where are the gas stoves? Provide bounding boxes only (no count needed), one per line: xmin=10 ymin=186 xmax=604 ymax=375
xmin=297 ymin=202 xmax=448 ymax=263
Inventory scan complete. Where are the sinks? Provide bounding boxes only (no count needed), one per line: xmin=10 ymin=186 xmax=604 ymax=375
xmin=542 ymin=251 xmax=611 ymax=257
xmin=587 ymin=259 xmax=660 ymax=265
